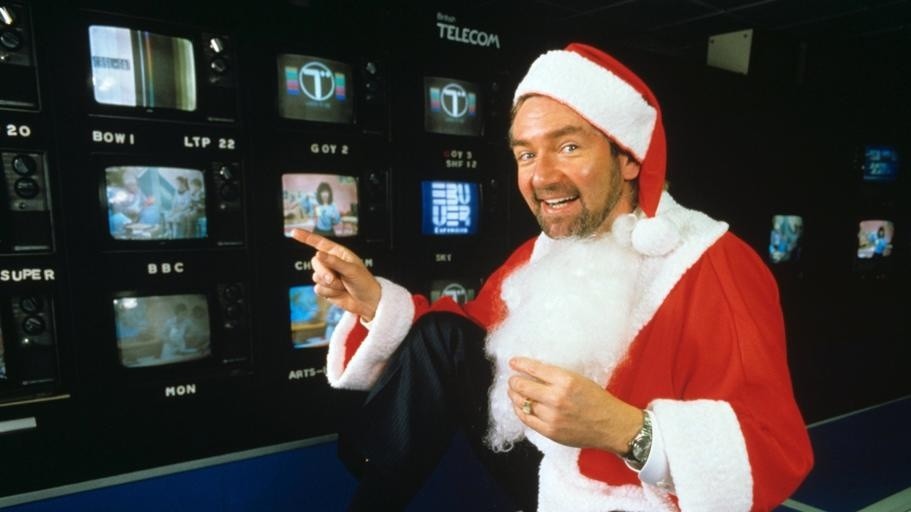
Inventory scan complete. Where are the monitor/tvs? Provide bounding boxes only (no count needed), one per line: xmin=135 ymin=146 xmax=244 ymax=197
xmin=0 ymin=0 xmax=66 ymax=143
xmin=1 ymin=139 xmax=66 ymax=258
xmin=247 ymin=137 xmax=392 ymax=249
xmin=247 ymin=37 xmax=386 ymax=136
xmin=405 ymin=63 xmax=485 ymax=140
xmin=81 ymin=249 xmax=253 ymax=402
xmin=857 ymin=220 xmax=895 ymax=259
xmin=400 ymin=246 xmax=484 ymax=304
xmin=67 ymin=6 xmax=240 ymax=118
xmin=254 ymin=250 xmax=395 ymax=386
xmin=66 ymin=118 xmax=246 ymax=246
xmin=0 ymin=260 xmax=77 ymax=407
xmin=862 ymin=148 xmax=900 ymax=181
xmin=768 ymin=214 xmax=804 ymax=264
xmin=407 ymin=143 xmax=485 ymax=243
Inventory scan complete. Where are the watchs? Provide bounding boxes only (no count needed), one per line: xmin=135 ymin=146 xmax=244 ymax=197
xmin=626 ymin=410 xmax=652 ymax=466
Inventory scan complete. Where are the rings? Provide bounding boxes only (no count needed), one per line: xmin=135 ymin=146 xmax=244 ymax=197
xmin=522 ymin=397 xmax=534 ymax=416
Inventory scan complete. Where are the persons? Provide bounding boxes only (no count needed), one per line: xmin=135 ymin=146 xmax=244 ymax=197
xmin=312 ymin=182 xmax=341 ymax=235
xmin=290 ymin=44 xmax=815 ymax=512
xmin=325 ymin=304 xmax=344 ymax=340
xmin=869 ymin=226 xmax=887 ymax=258
xmin=159 ymin=305 xmax=209 ymax=356
xmin=109 ymin=168 xmax=205 ymax=238
xmin=291 ymin=291 xmax=319 ymax=323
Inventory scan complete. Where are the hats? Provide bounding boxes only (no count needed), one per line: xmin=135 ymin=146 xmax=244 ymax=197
xmin=511 ymin=42 xmax=683 ymax=255
